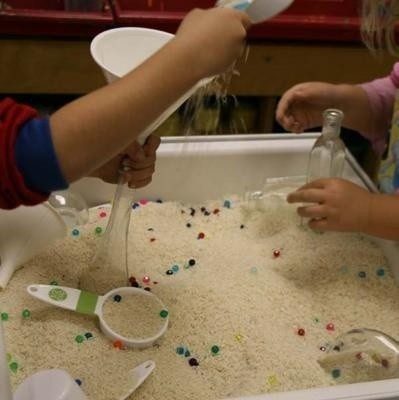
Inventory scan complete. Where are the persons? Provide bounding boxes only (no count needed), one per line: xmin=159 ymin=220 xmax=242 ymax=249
xmin=273 ymin=1 xmax=399 ymax=242
xmin=0 ymin=5 xmax=253 ymax=211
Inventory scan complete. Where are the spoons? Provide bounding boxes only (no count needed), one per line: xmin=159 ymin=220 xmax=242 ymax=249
xmin=26 ymin=283 xmax=170 ymax=345
xmin=11 ymin=360 xmax=155 ymax=400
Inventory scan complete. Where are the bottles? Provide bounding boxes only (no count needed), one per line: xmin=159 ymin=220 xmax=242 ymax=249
xmin=300 ymin=108 xmax=346 ymax=228
